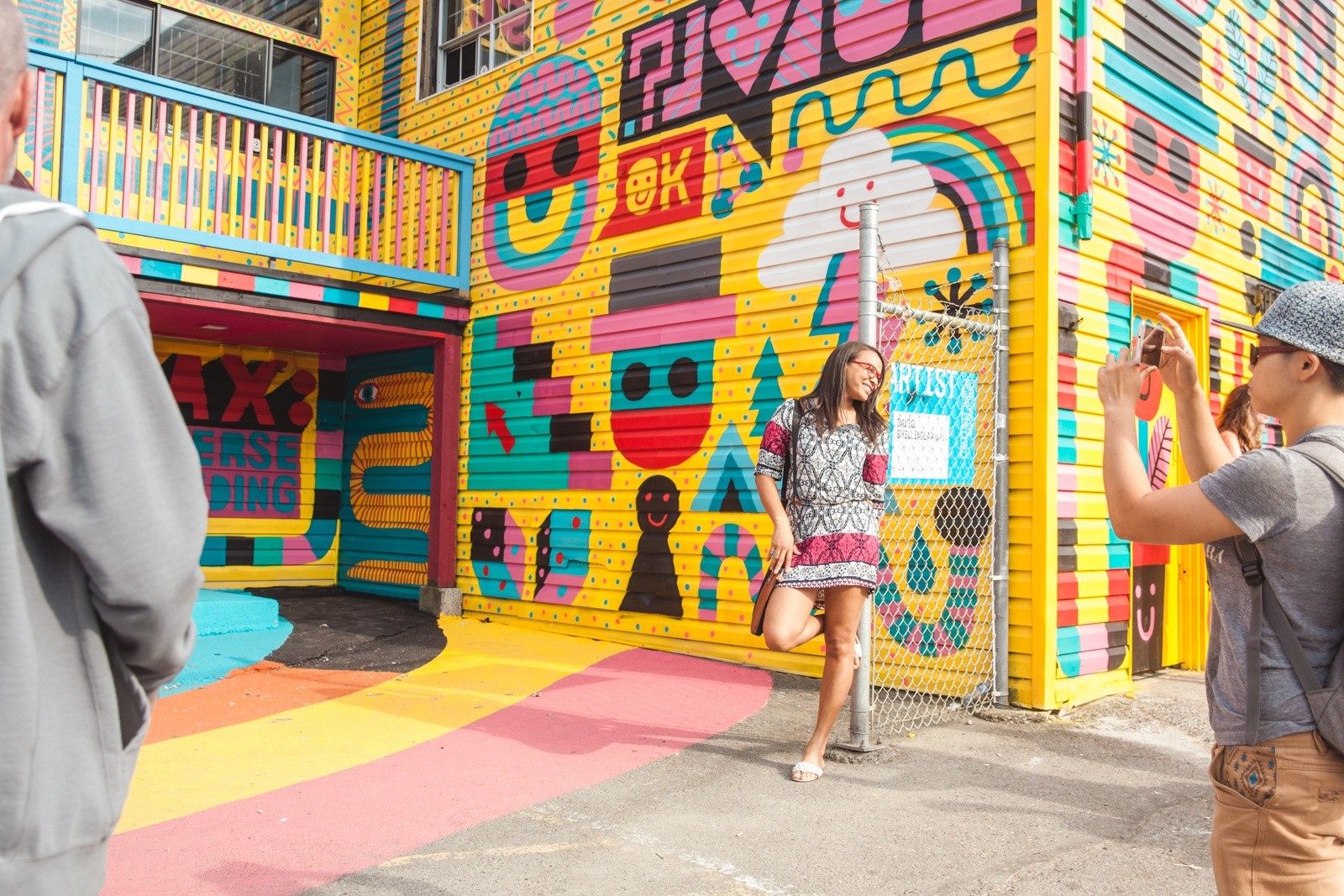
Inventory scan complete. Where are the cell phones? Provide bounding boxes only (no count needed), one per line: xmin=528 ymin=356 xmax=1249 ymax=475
xmin=1138 ymin=327 xmax=1167 ymax=369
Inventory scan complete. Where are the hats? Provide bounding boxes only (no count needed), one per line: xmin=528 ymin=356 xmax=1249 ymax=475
xmin=1255 ymin=280 xmax=1344 ymax=366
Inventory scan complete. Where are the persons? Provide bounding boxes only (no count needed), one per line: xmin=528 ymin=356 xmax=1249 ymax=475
xmin=1094 ymin=278 xmax=1344 ymax=895
xmin=753 ymin=341 xmax=891 ymax=783
xmin=1215 ymin=383 xmax=1270 ymax=459
xmin=1 ymin=1 xmax=209 ymax=896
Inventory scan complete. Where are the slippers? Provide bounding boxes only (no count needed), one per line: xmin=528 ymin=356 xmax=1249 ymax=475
xmin=791 ymin=760 xmax=825 ymax=782
xmin=853 ymin=633 xmax=862 ymax=667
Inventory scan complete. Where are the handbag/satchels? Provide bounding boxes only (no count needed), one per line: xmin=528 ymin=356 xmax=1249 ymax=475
xmin=750 ymin=558 xmax=783 ymax=636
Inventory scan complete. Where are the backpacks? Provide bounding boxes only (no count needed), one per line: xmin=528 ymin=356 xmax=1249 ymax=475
xmin=1232 ymin=437 xmax=1344 ymax=757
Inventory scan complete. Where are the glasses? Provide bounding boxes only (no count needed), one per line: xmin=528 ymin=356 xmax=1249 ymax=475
xmin=847 ymin=360 xmax=883 ymax=382
xmin=1249 ymin=342 xmax=1335 ymax=376
